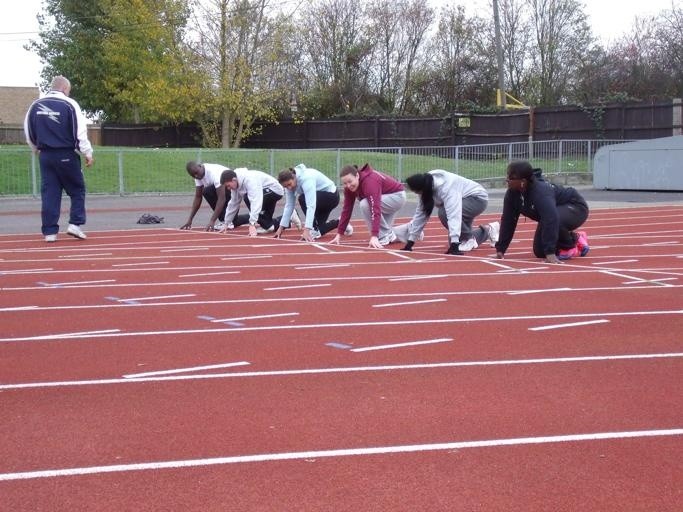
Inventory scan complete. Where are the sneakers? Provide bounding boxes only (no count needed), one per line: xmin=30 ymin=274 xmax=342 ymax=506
xmin=45 ymin=234 xmax=57 ymax=241
xmin=67 ymin=224 xmax=87 ymax=239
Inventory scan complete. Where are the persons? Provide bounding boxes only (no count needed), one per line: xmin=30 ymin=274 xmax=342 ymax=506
xmin=271 ymin=163 xmax=354 ymax=243
xmin=216 ymin=165 xmax=303 ymax=238
xmin=399 ymin=168 xmax=500 ymax=255
xmin=23 ymin=75 xmax=95 ymax=244
xmin=328 ymin=162 xmax=425 ymax=250
xmin=179 ymin=159 xmax=254 ymax=232
xmin=487 ymin=159 xmax=590 ymax=266
